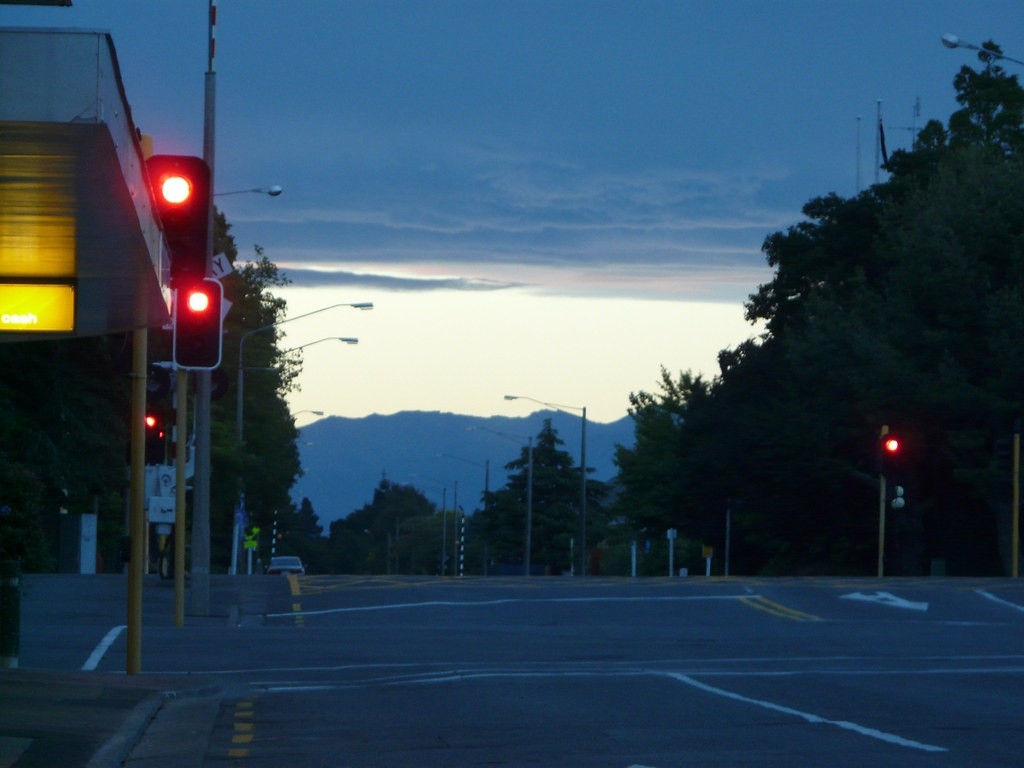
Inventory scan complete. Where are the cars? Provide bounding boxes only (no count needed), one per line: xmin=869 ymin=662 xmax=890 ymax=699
xmin=264 ymin=556 xmax=307 ymax=576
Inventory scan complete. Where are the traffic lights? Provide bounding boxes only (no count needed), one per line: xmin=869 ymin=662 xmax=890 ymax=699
xmin=881 ymin=432 xmax=903 ymax=480
xmin=173 ymin=274 xmax=224 ymax=370
xmin=142 ymin=155 xmax=210 ymax=282
xmin=144 ymin=410 xmax=166 ymax=448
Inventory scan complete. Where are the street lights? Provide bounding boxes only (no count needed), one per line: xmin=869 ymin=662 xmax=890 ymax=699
xmin=504 ymin=393 xmax=588 ymax=578
xmin=435 ymin=453 xmax=489 ymax=576
xmin=193 ymin=182 xmax=287 ymax=579
xmin=285 ymin=409 xmax=324 ymax=425
xmin=230 ymin=301 xmax=376 ymax=578
xmin=465 ymin=425 xmax=533 ymax=577
xmin=264 ymin=336 xmax=359 ymax=372
